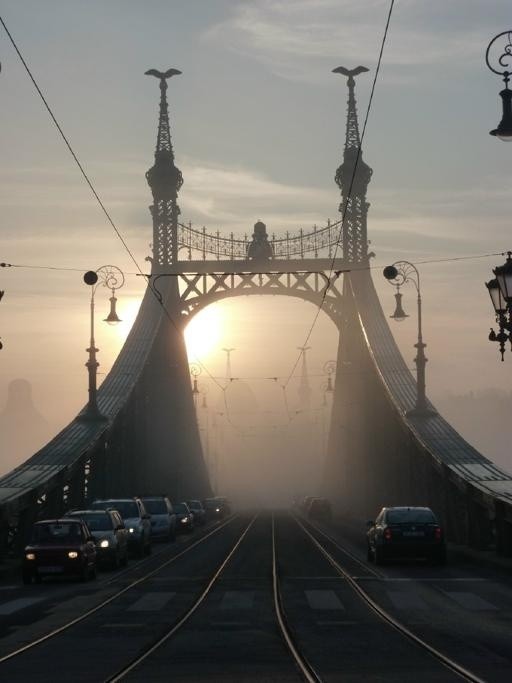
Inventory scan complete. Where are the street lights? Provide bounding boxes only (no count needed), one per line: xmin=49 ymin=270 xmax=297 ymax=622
xmin=76 ymin=264 xmax=124 ymax=422
xmin=486 ymin=28 xmax=511 ymax=363
xmin=387 ymin=261 xmax=437 ymax=418
xmin=296 ymin=361 xmax=339 ymax=463
xmin=190 ymin=364 xmax=224 ymax=497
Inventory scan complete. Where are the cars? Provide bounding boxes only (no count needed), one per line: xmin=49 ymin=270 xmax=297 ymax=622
xmin=289 ymin=494 xmax=332 ymax=519
xmin=24 ymin=519 xmax=97 ymax=582
xmin=366 ymin=506 xmax=447 ymax=566
xmin=174 ymin=495 xmax=230 ymax=534
xmin=62 ymin=505 xmax=129 ymax=568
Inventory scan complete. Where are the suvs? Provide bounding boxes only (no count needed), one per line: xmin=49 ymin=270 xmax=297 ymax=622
xmin=90 ymin=496 xmax=152 ymax=560
xmin=140 ymin=496 xmax=177 ymax=541
